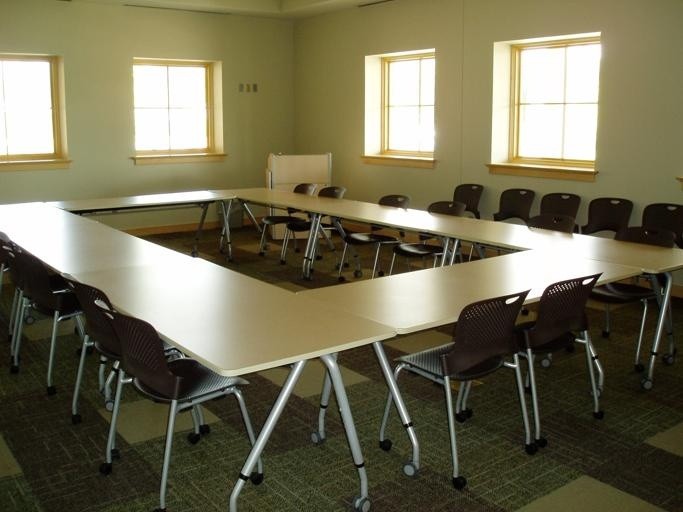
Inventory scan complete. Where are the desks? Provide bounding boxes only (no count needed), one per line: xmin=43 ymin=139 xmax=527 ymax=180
xmin=1 ymin=184 xmax=242 ymax=327
xmin=68 ymin=257 xmax=398 ymax=509
xmin=236 ymin=183 xmax=683 ymax=390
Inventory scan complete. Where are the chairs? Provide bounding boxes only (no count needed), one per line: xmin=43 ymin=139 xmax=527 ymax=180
xmin=99 ymin=309 xmax=264 ymax=511
xmin=2 ymin=238 xmax=116 ymax=426
xmin=462 ymin=273 xmax=605 ymax=449
xmin=378 ymin=290 xmax=540 ymax=491
xmin=259 ymin=181 xmax=683 ymax=374
